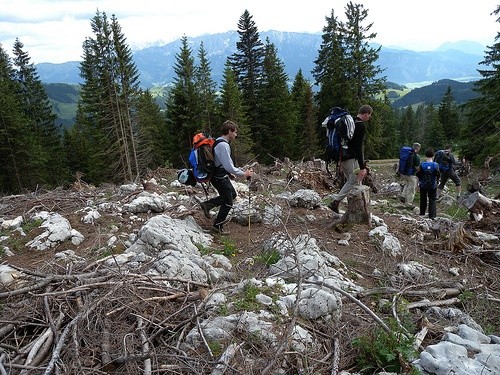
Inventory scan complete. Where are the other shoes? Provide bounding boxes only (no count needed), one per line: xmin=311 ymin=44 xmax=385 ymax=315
xmin=401 ymin=197 xmax=406 ymax=203
xmin=326 ymin=200 xmax=339 ymax=213
xmin=211 ymin=226 xmax=231 ymax=235
xmin=201 ymin=202 xmax=211 ymax=219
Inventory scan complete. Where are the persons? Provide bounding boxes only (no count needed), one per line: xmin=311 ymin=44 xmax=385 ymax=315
xmin=328 ymin=105 xmax=373 ymax=214
xmin=416 ymin=148 xmax=440 ymax=220
xmin=400 ymin=143 xmax=421 ymax=210
xmin=199 ymin=120 xmax=252 ymax=235
xmin=438 ymin=144 xmax=461 ymax=199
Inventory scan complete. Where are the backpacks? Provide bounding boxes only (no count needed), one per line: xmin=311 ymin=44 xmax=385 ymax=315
xmin=189 ymin=132 xmax=233 ymax=183
xmin=433 ymin=150 xmax=450 ymax=173
xmin=399 ymin=147 xmax=415 ymax=175
xmin=322 ymin=107 xmax=355 ymax=162
xmin=416 ymin=162 xmax=437 ymax=189
